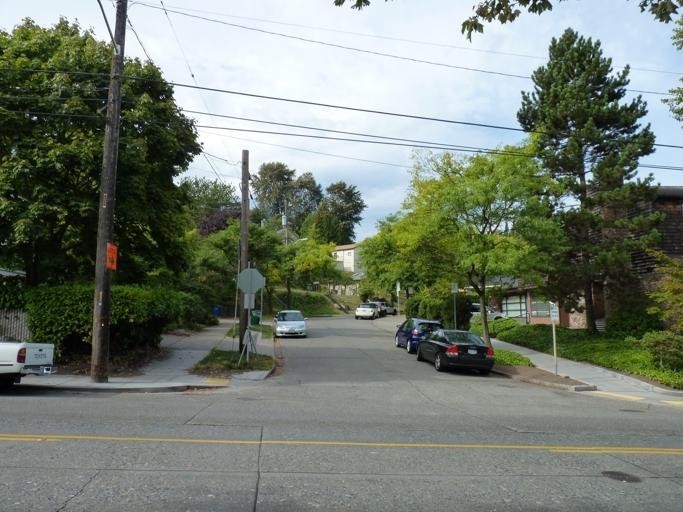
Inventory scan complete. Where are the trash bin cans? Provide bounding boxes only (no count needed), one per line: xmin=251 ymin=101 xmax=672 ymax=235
xmin=213 ymin=305 xmax=221 ymax=316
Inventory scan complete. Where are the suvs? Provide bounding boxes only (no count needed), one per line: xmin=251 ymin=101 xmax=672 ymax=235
xmin=369 ymin=301 xmax=398 ymax=316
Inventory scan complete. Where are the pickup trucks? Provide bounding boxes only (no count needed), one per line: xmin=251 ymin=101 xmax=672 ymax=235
xmin=0 ymin=341 xmax=55 ymax=385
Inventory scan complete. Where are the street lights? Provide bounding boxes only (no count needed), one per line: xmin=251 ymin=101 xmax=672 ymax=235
xmin=281 ymin=216 xmax=288 ymax=246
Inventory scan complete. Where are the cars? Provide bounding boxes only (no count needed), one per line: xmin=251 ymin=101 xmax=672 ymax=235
xmin=416 ymin=330 xmax=495 ymax=373
xmin=395 ymin=318 xmax=444 ymax=352
xmin=272 ymin=308 xmax=308 ymax=338
xmin=470 ymin=302 xmax=506 ymax=324
xmin=356 ymin=302 xmax=380 ymax=320
